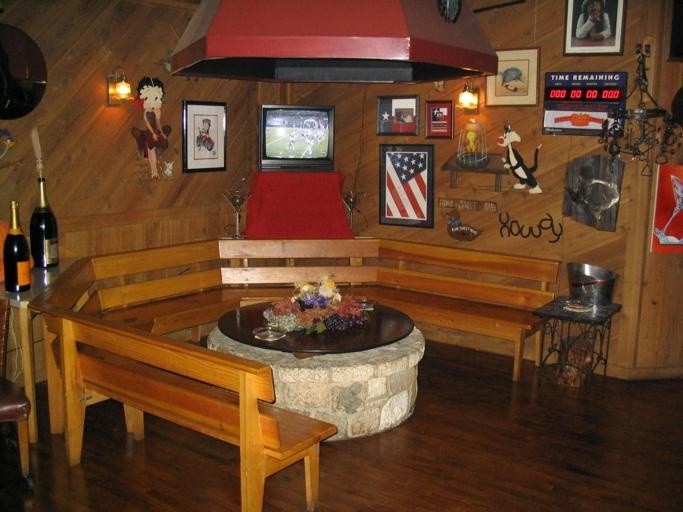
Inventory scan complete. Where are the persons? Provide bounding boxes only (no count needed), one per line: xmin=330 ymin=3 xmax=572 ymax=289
xmin=575 ymin=0 xmax=612 ymax=40
xmin=130 ymin=75 xmax=173 ymax=180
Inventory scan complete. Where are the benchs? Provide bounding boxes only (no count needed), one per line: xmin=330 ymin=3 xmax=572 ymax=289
xmin=27 ymin=239 xmax=560 ymax=512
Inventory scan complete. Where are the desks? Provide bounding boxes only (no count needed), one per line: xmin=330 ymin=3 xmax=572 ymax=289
xmin=207 ymin=301 xmax=424 ymax=444
xmin=531 ymin=296 xmax=622 ymax=406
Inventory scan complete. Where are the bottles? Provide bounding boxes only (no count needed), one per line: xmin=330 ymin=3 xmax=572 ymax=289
xmin=3 ymin=200 xmax=31 ymax=292
xmin=30 ymin=127 xmax=59 ymax=267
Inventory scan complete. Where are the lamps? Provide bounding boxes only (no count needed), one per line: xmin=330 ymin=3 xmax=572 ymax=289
xmin=106 ymin=71 xmax=133 ymax=106
xmin=457 ymin=81 xmax=479 ymax=115
xmin=169 ymin=0 xmax=496 ymax=85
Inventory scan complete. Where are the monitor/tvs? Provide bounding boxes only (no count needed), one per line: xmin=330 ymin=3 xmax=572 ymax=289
xmin=258 ymin=103 xmax=335 ymax=172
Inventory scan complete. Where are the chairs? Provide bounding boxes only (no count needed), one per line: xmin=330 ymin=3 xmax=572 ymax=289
xmin=0 ymin=376 xmax=32 ymax=477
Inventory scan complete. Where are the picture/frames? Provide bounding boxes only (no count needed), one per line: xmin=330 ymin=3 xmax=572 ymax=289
xmin=664 ymin=0 xmax=682 ymax=64
xmin=375 ymin=94 xmax=419 ymax=137
xmin=378 ymin=143 xmax=435 ymax=229
xmin=423 ymin=99 xmax=453 ymax=139
xmin=484 ymin=46 xmax=539 ymax=108
xmin=182 ymin=100 xmax=227 ymax=172
xmin=562 ymin=0 xmax=626 ymax=57
